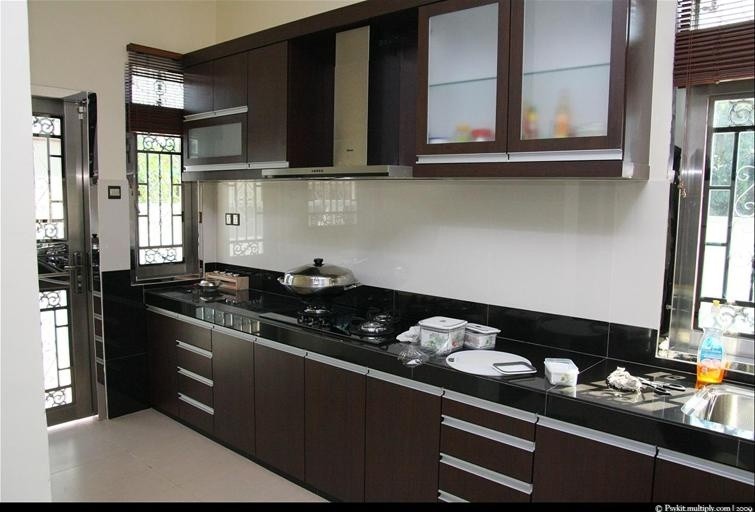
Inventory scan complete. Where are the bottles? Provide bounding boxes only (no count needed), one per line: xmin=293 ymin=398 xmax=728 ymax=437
xmin=526 ymin=106 xmax=540 ymax=141
xmin=553 ymin=92 xmax=570 ymax=139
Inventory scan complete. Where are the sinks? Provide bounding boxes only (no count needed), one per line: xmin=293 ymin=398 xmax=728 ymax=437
xmin=681 ymin=382 xmax=754 ymax=439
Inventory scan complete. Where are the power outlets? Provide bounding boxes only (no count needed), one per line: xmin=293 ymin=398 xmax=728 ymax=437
xmin=225 ymin=212 xmax=232 ymax=225
xmin=231 ymin=214 xmax=239 ymax=225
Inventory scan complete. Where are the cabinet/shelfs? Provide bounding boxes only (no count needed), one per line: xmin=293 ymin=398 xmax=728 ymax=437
xmin=438 ymin=388 xmax=537 ymax=505
xmin=412 ymin=0 xmax=656 ymax=182
xmin=180 ymin=22 xmax=290 ymax=180
xmin=654 ymin=445 xmax=755 ymax=506
xmin=254 ymin=337 xmax=305 ymax=482
xmin=530 ymin=414 xmax=657 ymax=502
xmin=212 ymin=323 xmax=256 ymax=460
xmin=144 ymin=304 xmax=177 ymax=420
xmin=366 ymin=368 xmax=444 ymax=504
xmin=289 ymin=1 xmax=418 ymax=180
xmin=175 ymin=314 xmax=214 ymax=438
xmin=306 ymin=352 xmax=365 ymax=503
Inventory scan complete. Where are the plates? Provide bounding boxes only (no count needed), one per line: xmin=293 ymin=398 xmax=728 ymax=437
xmin=445 ymin=348 xmax=534 ymax=378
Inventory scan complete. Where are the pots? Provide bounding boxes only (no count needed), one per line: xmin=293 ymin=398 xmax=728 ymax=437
xmin=276 ymin=258 xmax=360 ymax=303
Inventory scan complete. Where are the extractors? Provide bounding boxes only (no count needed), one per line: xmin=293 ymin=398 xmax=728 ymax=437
xmin=261 ymin=23 xmax=414 ymax=182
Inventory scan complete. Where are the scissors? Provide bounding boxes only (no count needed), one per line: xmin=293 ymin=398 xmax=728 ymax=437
xmin=638 ymin=378 xmax=686 ymax=396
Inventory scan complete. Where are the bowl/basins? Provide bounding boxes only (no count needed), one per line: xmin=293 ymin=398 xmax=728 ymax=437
xmin=194 ymin=281 xmax=220 ymax=292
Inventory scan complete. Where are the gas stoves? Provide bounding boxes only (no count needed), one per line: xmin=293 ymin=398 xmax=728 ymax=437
xmin=258 ymin=300 xmax=411 ymax=346
